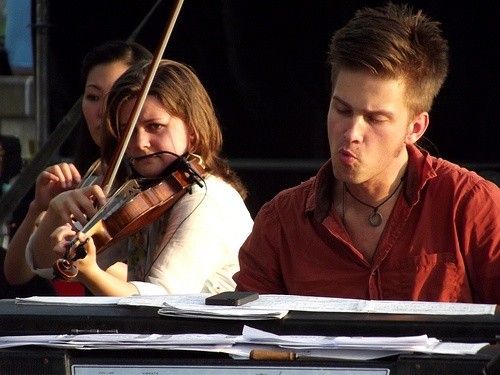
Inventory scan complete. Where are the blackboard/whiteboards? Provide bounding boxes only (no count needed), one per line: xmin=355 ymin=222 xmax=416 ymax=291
xmin=31 ymin=0 xmax=500 ymax=174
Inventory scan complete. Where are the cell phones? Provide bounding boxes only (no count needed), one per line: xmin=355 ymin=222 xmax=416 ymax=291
xmin=206 ymin=292 xmax=259 ymax=306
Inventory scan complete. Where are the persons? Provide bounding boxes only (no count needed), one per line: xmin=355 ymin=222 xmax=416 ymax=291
xmin=232 ymin=4 xmax=500 ymax=303
xmin=2 ymin=41 xmax=157 ymax=297
xmin=0 ymin=134 xmax=29 ymax=252
xmin=24 ymin=59 xmax=255 ymax=297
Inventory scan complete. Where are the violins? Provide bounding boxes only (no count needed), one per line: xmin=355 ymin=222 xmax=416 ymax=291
xmin=52 ymin=152 xmax=208 ymax=284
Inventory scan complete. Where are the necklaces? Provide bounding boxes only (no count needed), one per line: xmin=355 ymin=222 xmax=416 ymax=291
xmin=341 ymin=172 xmax=407 ymax=227
